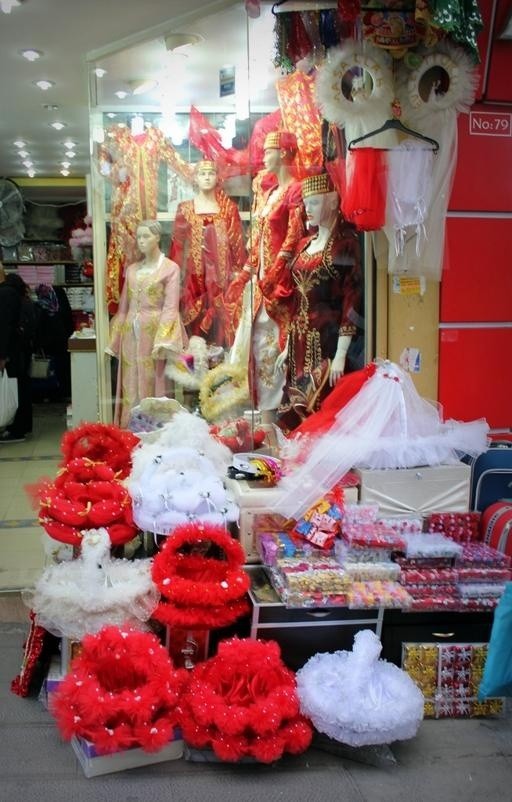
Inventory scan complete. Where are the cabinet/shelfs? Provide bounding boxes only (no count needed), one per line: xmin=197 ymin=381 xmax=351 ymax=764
xmin=1 ymin=260 xmax=94 ymax=286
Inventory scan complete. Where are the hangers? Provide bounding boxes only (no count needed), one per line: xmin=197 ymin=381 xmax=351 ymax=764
xmin=348 ymin=99 xmax=439 ymax=151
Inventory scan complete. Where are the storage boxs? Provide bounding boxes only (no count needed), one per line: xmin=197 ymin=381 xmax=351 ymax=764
xmin=380 ymin=609 xmax=494 ymax=670
xmin=354 ymin=463 xmax=471 ymax=518
xmin=204 ymin=563 xmax=384 ymax=672
xmin=225 ymin=478 xmax=359 ymax=563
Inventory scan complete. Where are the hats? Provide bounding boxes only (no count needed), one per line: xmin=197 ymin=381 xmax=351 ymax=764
xmin=262 ymin=130 xmax=299 ymax=152
xmin=194 ymin=159 xmax=218 ymax=173
xmin=300 ymin=173 xmax=337 ymax=199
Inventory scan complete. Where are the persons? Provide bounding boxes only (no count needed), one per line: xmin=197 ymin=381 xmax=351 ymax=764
xmin=106 ymin=219 xmax=188 ymax=429
xmin=274 ymin=175 xmax=365 ymax=428
xmin=0 ymin=272 xmax=75 ymax=443
xmin=170 ymin=160 xmax=247 ymax=350
xmin=224 ymin=132 xmax=307 ymax=434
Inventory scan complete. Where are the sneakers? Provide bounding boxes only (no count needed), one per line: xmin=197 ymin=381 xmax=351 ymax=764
xmin=0 ymin=424 xmax=34 ymax=443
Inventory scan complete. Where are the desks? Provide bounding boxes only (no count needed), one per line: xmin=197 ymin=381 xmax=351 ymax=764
xmin=67 ymin=333 xmax=98 ymax=427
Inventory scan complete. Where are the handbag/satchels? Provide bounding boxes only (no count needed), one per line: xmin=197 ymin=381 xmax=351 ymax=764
xmin=28 ymin=349 xmax=50 ymax=379
xmin=0 ymin=368 xmax=20 ymax=427
